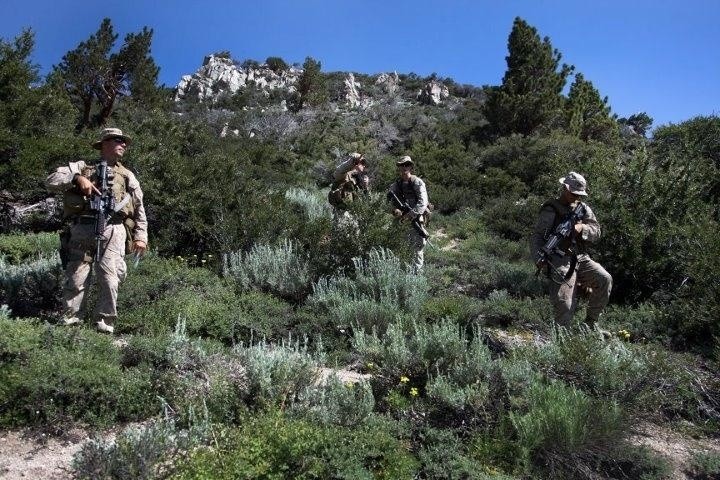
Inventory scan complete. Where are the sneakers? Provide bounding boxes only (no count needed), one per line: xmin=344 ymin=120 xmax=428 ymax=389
xmin=96 ymin=319 xmax=114 ymax=332
xmin=57 ymin=315 xmax=80 ymax=326
xmin=584 ymin=316 xmax=611 ymax=338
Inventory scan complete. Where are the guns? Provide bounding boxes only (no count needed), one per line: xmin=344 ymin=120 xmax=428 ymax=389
xmin=535 ymin=201 xmax=585 ymax=277
xmin=80 ymin=160 xmax=115 ymax=263
xmin=386 ymin=191 xmax=435 ymax=249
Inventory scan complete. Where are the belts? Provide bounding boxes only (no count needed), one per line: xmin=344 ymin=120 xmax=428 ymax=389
xmin=73 ymin=217 xmax=124 ymax=225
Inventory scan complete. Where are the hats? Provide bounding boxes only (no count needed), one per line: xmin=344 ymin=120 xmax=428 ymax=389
xmin=558 ymin=172 xmax=589 ymax=197
xmin=92 ymin=127 xmax=132 ymax=150
xmin=395 ymin=155 xmax=414 ymax=166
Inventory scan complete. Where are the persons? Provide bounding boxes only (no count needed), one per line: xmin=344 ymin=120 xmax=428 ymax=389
xmin=44 ymin=128 xmax=148 ymax=333
xmin=328 ymin=152 xmax=370 ymax=235
xmin=529 ymin=171 xmax=613 ymax=340
xmin=381 ymin=156 xmax=429 ymax=274
